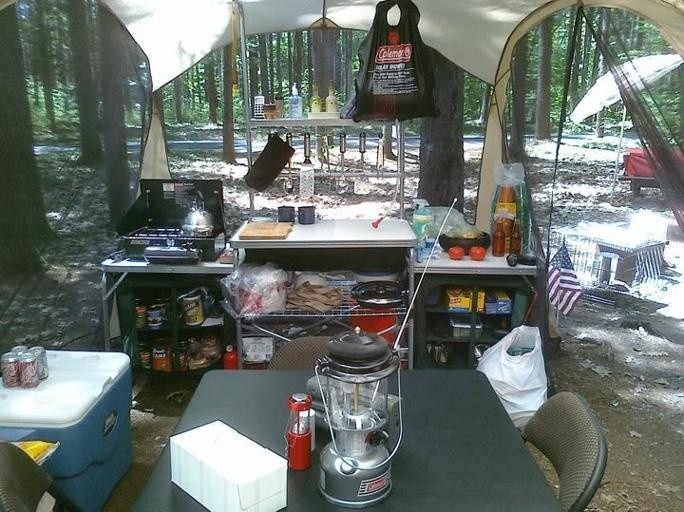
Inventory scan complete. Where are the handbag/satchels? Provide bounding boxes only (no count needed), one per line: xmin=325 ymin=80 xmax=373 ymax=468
xmin=476 ymin=325 xmax=548 ymax=431
xmin=352 ymin=0 xmax=441 ymax=122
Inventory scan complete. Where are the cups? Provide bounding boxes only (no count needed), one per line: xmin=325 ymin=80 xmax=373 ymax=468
xmin=297 ymin=206 xmax=316 ymax=224
xmin=278 ymin=206 xmax=295 ymax=223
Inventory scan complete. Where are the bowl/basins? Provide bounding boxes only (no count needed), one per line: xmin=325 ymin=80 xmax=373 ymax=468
xmin=440 ymin=231 xmax=489 ymax=254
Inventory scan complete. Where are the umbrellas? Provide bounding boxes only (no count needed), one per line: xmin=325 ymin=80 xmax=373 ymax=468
xmin=570 ymin=54 xmax=681 ymax=203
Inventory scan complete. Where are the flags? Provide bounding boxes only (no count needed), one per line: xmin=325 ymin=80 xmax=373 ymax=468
xmin=546 ymin=244 xmax=583 ymax=317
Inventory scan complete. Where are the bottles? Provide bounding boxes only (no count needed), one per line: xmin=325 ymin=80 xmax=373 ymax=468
xmin=326 ymin=80 xmax=337 ymax=113
xmin=510 ymin=219 xmax=524 ymax=257
xmin=492 ymin=220 xmax=505 ymax=257
xmin=310 ymin=81 xmax=323 ymax=113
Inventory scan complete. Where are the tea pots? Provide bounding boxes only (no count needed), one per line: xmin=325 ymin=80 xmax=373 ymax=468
xmin=183 ymin=190 xmax=214 ymax=234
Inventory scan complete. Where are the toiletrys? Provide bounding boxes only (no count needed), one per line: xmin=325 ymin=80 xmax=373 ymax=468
xmin=288 ymin=83 xmax=302 ymax=118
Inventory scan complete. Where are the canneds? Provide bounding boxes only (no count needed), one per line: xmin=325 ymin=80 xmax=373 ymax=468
xmin=28 ymin=346 xmax=49 ymax=381
xmin=18 ymin=353 xmax=39 ymax=389
xmin=135 ymin=297 xmax=170 ymax=328
xmin=11 ymin=346 xmax=28 ymax=356
xmin=0 ymin=352 xmax=21 ymax=388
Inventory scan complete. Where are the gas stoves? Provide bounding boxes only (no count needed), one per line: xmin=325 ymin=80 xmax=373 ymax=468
xmin=126 ymin=225 xmax=226 ymax=261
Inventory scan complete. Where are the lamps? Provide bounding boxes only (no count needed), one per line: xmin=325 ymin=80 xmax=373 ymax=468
xmin=312 ymin=327 xmax=409 ymax=508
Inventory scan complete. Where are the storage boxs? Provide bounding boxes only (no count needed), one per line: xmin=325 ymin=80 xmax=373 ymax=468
xmin=0 ymin=349 xmax=132 ymax=511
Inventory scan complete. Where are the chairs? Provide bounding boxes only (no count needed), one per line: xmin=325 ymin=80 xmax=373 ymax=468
xmin=518 ymin=390 xmax=610 ymax=512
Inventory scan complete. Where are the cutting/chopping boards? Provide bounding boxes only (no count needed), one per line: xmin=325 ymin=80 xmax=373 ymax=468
xmin=239 ymin=219 xmax=294 ymax=240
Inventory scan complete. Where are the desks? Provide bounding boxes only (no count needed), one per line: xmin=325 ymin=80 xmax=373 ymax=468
xmin=128 ymin=369 xmax=567 ymax=512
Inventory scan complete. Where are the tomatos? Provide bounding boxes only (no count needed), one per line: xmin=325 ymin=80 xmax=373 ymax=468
xmin=469 ymin=245 xmax=485 ymax=261
xmin=448 ymin=246 xmax=464 ymax=260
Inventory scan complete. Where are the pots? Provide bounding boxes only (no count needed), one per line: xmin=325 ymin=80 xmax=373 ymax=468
xmin=351 ymin=280 xmax=407 ymax=309
